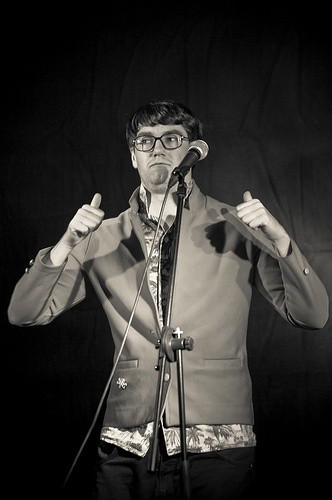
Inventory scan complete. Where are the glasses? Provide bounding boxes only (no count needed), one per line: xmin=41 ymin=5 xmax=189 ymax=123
xmin=132 ymin=133 xmax=190 ymax=152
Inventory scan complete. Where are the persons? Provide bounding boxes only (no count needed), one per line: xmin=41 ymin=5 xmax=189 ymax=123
xmin=9 ymin=99 xmax=330 ymax=500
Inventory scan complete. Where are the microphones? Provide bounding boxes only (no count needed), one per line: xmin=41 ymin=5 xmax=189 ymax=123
xmin=168 ymin=141 xmax=209 ymax=189
